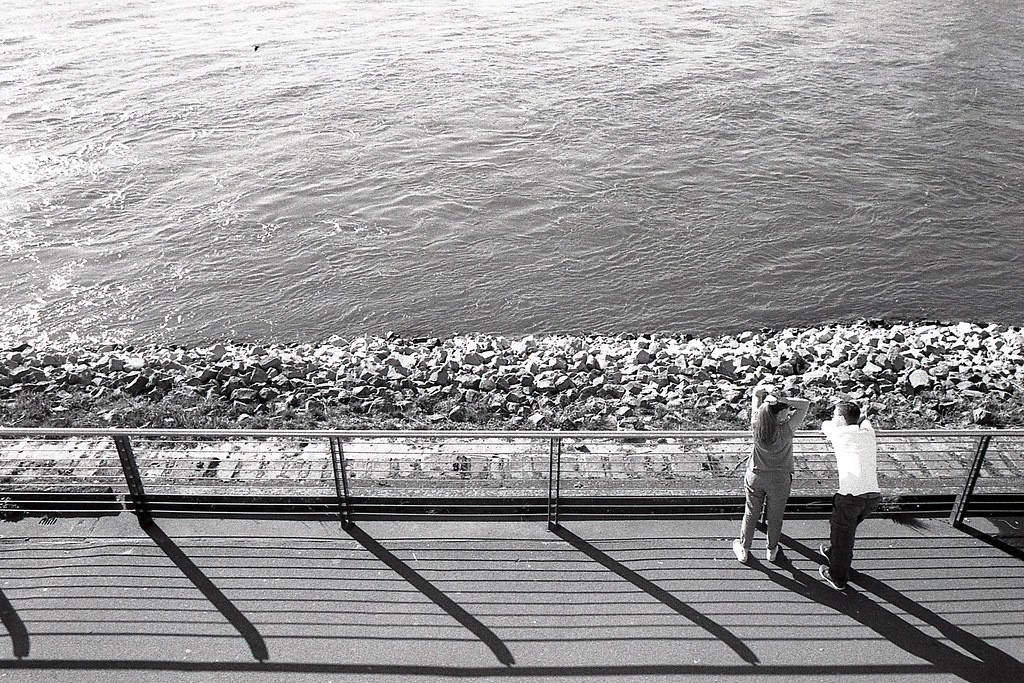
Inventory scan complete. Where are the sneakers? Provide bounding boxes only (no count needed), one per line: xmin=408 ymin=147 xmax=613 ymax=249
xmin=819 ymin=564 xmax=845 ymax=590
xmin=767 ymin=545 xmax=778 ymax=561
xmin=733 ymin=539 xmax=748 ymax=563
xmin=819 ymin=544 xmax=831 ymax=565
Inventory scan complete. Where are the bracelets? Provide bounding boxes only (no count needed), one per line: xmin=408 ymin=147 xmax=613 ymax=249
xmin=777 ymin=398 xmax=778 ymax=404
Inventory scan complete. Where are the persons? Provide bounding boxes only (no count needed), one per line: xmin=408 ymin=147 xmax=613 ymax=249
xmin=819 ymin=401 xmax=880 ymax=592
xmin=733 ymin=384 xmax=809 ymax=563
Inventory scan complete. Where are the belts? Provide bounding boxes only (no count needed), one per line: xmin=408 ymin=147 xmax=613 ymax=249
xmin=857 ymin=493 xmax=882 ymax=498
